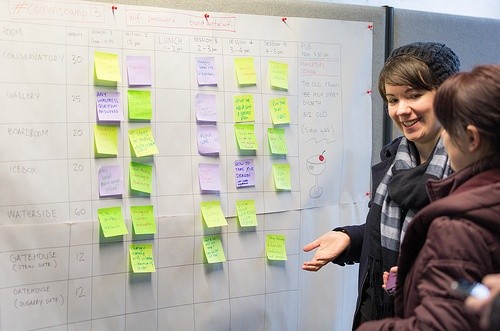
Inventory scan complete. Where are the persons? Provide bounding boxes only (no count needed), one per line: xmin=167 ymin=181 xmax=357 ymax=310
xmin=301 ymin=42 xmax=460 ymax=331
xmin=355 ymin=64 xmax=500 ymax=331
